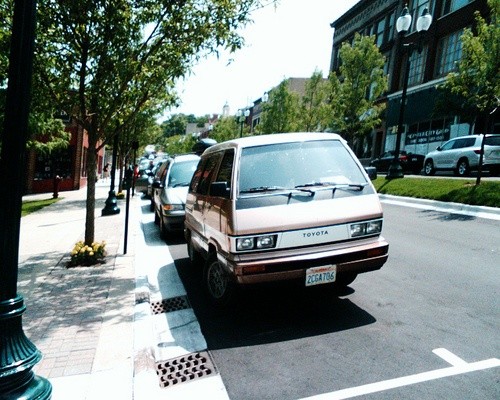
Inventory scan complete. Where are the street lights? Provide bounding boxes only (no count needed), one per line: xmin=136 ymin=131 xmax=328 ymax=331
xmin=383 ymin=4 xmax=432 ymax=180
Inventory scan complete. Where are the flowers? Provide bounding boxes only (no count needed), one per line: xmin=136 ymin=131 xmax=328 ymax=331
xmin=69 ymin=240 xmax=106 ymax=265
xmin=115 ymin=192 xmax=125 ymax=200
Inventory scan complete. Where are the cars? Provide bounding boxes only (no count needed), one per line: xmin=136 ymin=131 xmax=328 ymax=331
xmin=369 ymin=149 xmax=425 ymax=173
xmin=135 ymin=155 xmax=201 ymax=240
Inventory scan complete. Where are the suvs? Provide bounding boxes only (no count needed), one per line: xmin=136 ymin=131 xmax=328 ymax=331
xmin=422 ymin=134 xmax=500 ymax=177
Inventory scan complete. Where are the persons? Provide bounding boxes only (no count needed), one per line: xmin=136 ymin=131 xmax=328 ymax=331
xmin=103 ymin=163 xmax=110 ymax=183
xmin=126 ymin=165 xmax=133 ymax=188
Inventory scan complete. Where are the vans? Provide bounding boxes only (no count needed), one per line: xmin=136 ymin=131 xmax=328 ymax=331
xmin=186 ymin=132 xmax=388 ymax=301
